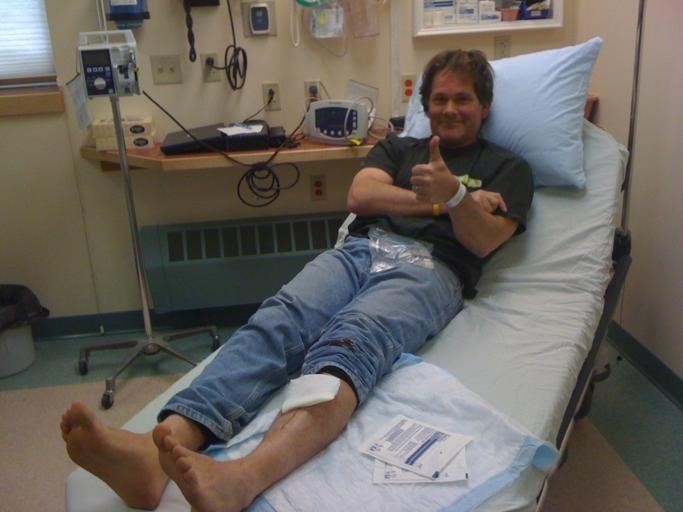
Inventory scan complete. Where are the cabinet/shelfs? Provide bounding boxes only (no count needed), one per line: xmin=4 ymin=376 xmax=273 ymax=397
xmin=409 ymin=0 xmax=566 ymax=41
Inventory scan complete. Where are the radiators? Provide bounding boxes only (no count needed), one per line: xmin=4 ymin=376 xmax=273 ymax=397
xmin=138 ymin=210 xmax=349 ymax=315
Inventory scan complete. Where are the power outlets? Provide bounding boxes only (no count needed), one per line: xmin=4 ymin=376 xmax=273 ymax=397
xmin=259 ymin=80 xmax=281 ymax=112
xmin=198 ymin=52 xmax=222 ymax=83
xmin=302 ymin=79 xmax=322 ymax=107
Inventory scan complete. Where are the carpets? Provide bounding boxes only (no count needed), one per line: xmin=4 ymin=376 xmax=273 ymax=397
xmin=0 ymin=371 xmax=663 ymax=511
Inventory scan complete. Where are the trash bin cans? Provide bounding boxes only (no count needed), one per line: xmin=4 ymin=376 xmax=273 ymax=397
xmin=0 ymin=284 xmax=49 ymax=378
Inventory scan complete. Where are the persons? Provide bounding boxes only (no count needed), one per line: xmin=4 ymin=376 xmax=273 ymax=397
xmin=59 ymin=49 xmax=533 ymax=512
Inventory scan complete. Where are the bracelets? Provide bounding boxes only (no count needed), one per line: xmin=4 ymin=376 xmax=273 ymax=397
xmin=432 ymin=204 xmax=440 ymax=216
xmin=445 ymin=181 xmax=466 ymax=209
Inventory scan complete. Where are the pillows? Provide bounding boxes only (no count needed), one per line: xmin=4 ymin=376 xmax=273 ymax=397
xmin=401 ymin=34 xmax=609 ymax=194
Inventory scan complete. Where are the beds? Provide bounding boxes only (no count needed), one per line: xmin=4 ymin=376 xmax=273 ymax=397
xmin=62 ymin=109 xmax=631 ymax=512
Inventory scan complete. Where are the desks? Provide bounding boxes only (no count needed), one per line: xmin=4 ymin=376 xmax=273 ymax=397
xmin=80 ymin=126 xmax=409 ymax=173
